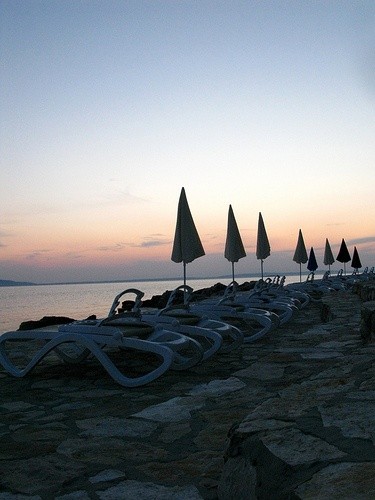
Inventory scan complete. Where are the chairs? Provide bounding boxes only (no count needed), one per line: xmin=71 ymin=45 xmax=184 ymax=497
xmin=1 ymin=267 xmax=375 ymax=390
